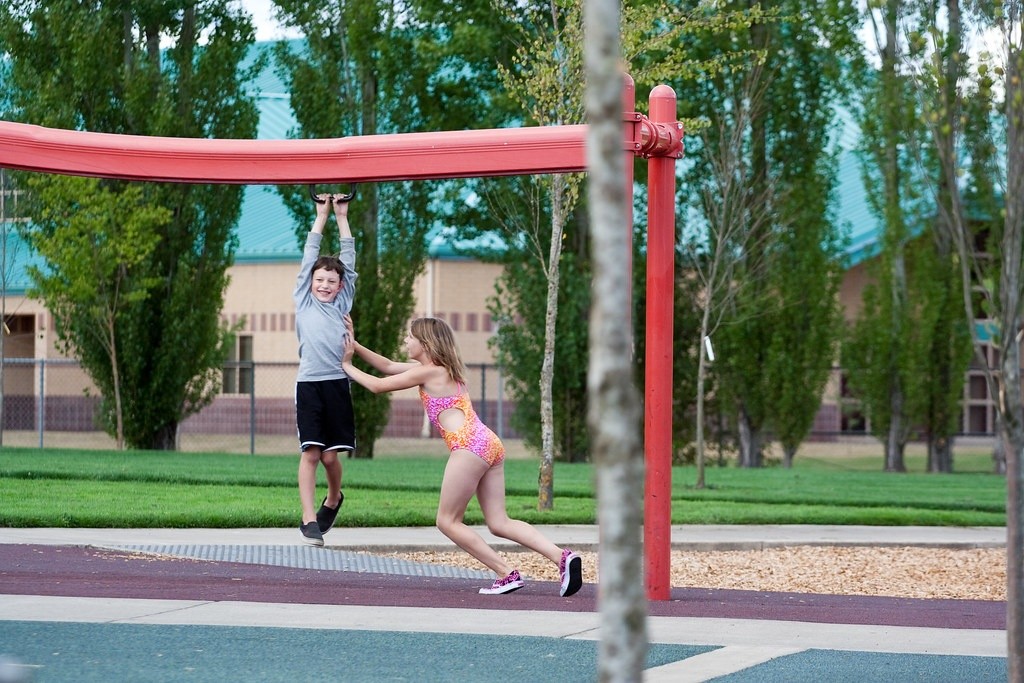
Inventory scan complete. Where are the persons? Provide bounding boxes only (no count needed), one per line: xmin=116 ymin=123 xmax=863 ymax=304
xmin=342 ymin=313 xmax=583 ymax=597
xmin=294 ymin=193 xmax=358 ymax=546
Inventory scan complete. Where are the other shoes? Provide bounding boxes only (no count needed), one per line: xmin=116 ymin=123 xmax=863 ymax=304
xmin=479 ymin=569 xmax=524 ymax=595
xmin=314 ymin=490 xmax=344 ymax=534
xmin=559 ymin=550 xmax=582 ymax=597
xmin=300 ymin=519 xmax=325 ymax=546
